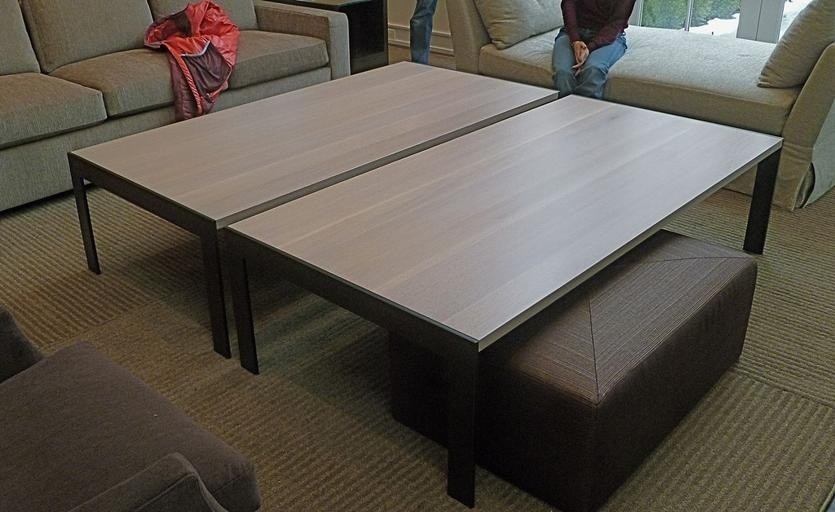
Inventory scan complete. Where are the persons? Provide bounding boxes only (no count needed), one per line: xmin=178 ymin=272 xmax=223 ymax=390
xmin=550 ymin=0 xmax=637 ymax=100
xmin=406 ymin=1 xmax=442 ymax=65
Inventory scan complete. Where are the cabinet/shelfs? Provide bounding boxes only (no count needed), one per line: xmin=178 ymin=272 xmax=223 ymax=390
xmin=280 ymin=0 xmax=390 ymax=73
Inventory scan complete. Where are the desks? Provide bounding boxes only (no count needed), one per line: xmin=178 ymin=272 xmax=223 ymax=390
xmin=217 ymin=90 xmax=785 ymax=507
xmin=63 ymin=59 xmax=561 ymax=362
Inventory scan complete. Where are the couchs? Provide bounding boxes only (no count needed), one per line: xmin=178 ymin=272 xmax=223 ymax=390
xmin=1 ymin=301 xmax=265 ymax=512
xmin=440 ymin=1 xmax=835 ymax=215
xmin=1 ymin=2 xmax=351 ymax=221
xmin=385 ymin=228 xmax=762 ymax=508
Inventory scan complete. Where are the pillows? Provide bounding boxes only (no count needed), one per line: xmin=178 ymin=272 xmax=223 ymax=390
xmin=757 ymin=0 xmax=834 ymax=91
xmin=470 ymin=2 xmax=574 ymax=50
